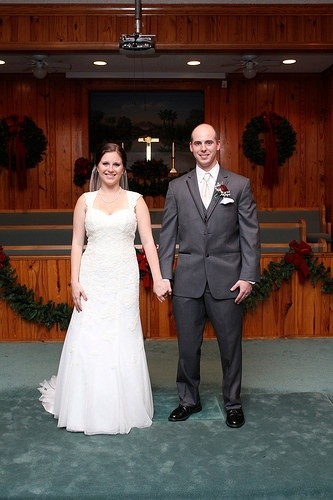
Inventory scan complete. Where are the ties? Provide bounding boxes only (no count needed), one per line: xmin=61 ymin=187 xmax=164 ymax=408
xmin=202 ymin=173 xmax=212 ymax=206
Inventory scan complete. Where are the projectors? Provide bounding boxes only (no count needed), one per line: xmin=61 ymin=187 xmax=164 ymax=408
xmin=119 ymin=34 xmax=155 ymax=57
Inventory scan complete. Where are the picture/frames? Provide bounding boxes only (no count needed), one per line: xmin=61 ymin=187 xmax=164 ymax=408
xmin=87 ymin=88 xmax=206 ymax=172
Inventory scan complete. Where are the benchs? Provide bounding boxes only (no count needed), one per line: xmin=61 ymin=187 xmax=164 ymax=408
xmin=0 ymin=206 xmax=333 ymax=255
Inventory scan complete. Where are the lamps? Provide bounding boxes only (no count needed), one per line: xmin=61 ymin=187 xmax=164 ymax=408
xmin=31 ymin=55 xmax=49 ymax=80
xmin=240 ymin=55 xmax=258 ymax=80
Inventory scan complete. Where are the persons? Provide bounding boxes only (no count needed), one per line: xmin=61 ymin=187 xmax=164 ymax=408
xmin=158 ymin=124 xmax=260 ymax=428
xmin=38 ymin=142 xmax=169 ymax=436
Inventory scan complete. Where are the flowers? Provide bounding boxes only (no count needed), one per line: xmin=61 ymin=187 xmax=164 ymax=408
xmin=215 ymin=181 xmax=231 ymax=197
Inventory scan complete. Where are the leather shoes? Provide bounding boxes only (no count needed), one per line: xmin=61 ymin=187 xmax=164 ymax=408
xmin=225 ymin=408 xmax=245 ymax=428
xmin=167 ymin=404 xmax=201 ymax=422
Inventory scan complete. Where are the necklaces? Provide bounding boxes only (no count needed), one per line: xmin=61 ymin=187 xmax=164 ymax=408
xmin=98 ymin=187 xmax=122 ymax=203
